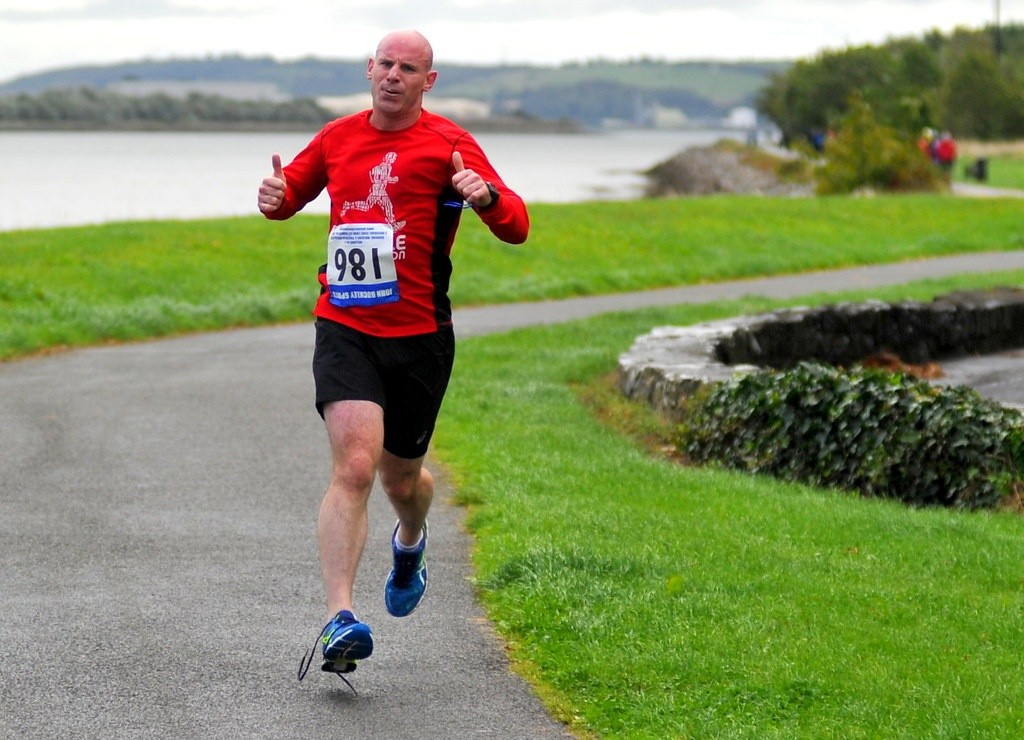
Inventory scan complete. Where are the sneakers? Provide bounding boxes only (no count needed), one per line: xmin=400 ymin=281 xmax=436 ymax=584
xmin=384 ymin=516 xmax=428 ymax=616
xmin=321 ymin=611 xmax=374 ymax=674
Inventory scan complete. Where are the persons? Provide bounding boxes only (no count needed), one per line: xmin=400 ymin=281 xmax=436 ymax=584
xmin=255 ymin=26 xmax=530 ymax=674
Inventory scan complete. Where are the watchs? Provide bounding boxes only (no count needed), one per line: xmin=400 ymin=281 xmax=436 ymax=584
xmin=473 ymin=181 xmax=500 ymax=211
